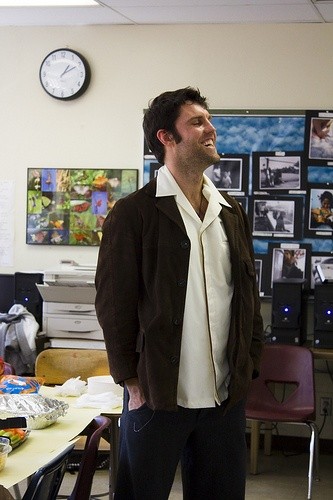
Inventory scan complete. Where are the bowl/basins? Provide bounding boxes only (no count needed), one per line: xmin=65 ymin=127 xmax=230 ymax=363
xmin=87 ymin=376 xmax=117 ymax=395
xmin=0 ymin=437 xmax=12 ymax=472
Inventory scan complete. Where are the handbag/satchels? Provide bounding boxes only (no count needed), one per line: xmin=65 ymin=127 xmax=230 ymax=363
xmin=0 ymin=393 xmax=68 ymax=430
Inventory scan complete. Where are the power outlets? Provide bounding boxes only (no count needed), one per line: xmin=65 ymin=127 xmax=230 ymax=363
xmin=320 ymin=398 xmax=333 ymax=417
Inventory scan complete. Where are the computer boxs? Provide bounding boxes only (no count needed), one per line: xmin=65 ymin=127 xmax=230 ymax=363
xmin=270 ymin=278 xmax=307 ymax=347
xmin=0 ymin=273 xmax=43 ymax=332
xmin=314 ymin=282 xmax=333 ymax=350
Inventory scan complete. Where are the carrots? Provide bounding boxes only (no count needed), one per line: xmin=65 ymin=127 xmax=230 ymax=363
xmin=6 ymin=428 xmax=26 ymax=439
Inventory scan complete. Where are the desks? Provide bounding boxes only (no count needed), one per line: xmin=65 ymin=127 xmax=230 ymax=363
xmin=0 ymin=387 xmax=124 ymax=489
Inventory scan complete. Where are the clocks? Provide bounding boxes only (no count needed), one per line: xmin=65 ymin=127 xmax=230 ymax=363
xmin=39 ymin=48 xmax=91 ymax=101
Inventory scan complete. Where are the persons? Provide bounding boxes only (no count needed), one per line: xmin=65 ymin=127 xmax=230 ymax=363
xmin=311 ymin=192 xmax=333 ymax=229
xmin=95 ymin=86 xmax=264 ymax=500
xmin=254 ymin=200 xmax=291 ymax=232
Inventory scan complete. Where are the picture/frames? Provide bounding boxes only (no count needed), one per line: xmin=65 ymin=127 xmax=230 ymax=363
xmin=26 ymin=167 xmax=139 ymax=246
xmin=304 ymin=110 xmax=333 ymax=166
xmin=304 ymin=182 xmax=333 ymax=239
xmin=250 ymin=196 xmax=303 ymax=240
xmin=254 ymin=242 xmax=333 ymax=299
xmin=212 ymin=151 xmax=308 ymax=195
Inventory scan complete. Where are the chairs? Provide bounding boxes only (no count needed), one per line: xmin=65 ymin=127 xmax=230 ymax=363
xmin=34 ymin=348 xmax=125 ymax=456
xmin=22 ymin=416 xmax=120 ymax=500
xmin=247 ymin=344 xmax=321 ymax=500
